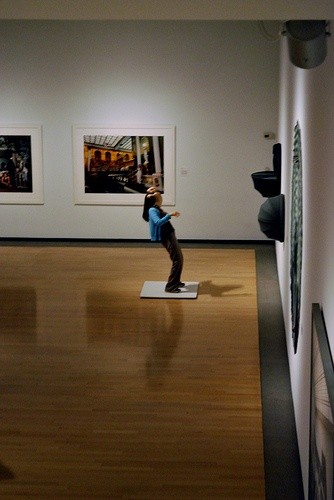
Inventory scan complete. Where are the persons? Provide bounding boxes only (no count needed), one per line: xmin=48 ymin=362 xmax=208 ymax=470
xmin=143 ymin=188 xmax=185 ymax=293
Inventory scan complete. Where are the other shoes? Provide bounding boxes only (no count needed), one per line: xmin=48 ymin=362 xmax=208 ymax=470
xmin=165 ymin=289 xmax=180 ymax=293
xmin=178 ymin=282 xmax=185 ymax=287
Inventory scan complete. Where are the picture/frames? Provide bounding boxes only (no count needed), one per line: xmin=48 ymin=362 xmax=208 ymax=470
xmin=0 ymin=124 xmax=44 ymax=204
xmin=71 ymin=124 xmax=177 ymax=207
xmin=307 ymin=302 xmax=333 ymax=500
